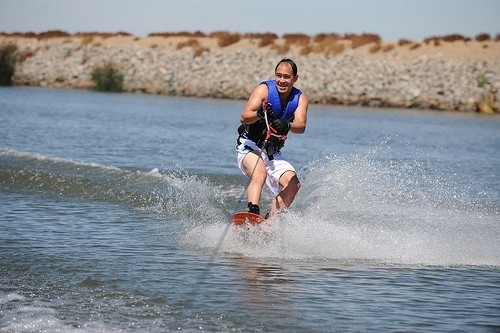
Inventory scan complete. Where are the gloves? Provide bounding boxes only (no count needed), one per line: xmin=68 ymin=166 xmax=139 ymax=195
xmin=271 ymin=119 xmax=290 ymax=135
xmin=257 ymin=102 xmax=275 ymax=120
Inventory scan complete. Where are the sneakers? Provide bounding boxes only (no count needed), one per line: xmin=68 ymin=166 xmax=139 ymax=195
xmin=248 ymin=202 xmax=259 ymax=216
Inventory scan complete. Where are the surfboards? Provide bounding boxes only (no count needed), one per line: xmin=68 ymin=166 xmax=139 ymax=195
xmin=232 ymin=212 xmax=263 ymax=225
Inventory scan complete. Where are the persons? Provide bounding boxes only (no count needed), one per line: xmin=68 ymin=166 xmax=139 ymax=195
xmin=236 ymin=59 xmax=308 ymax=220
